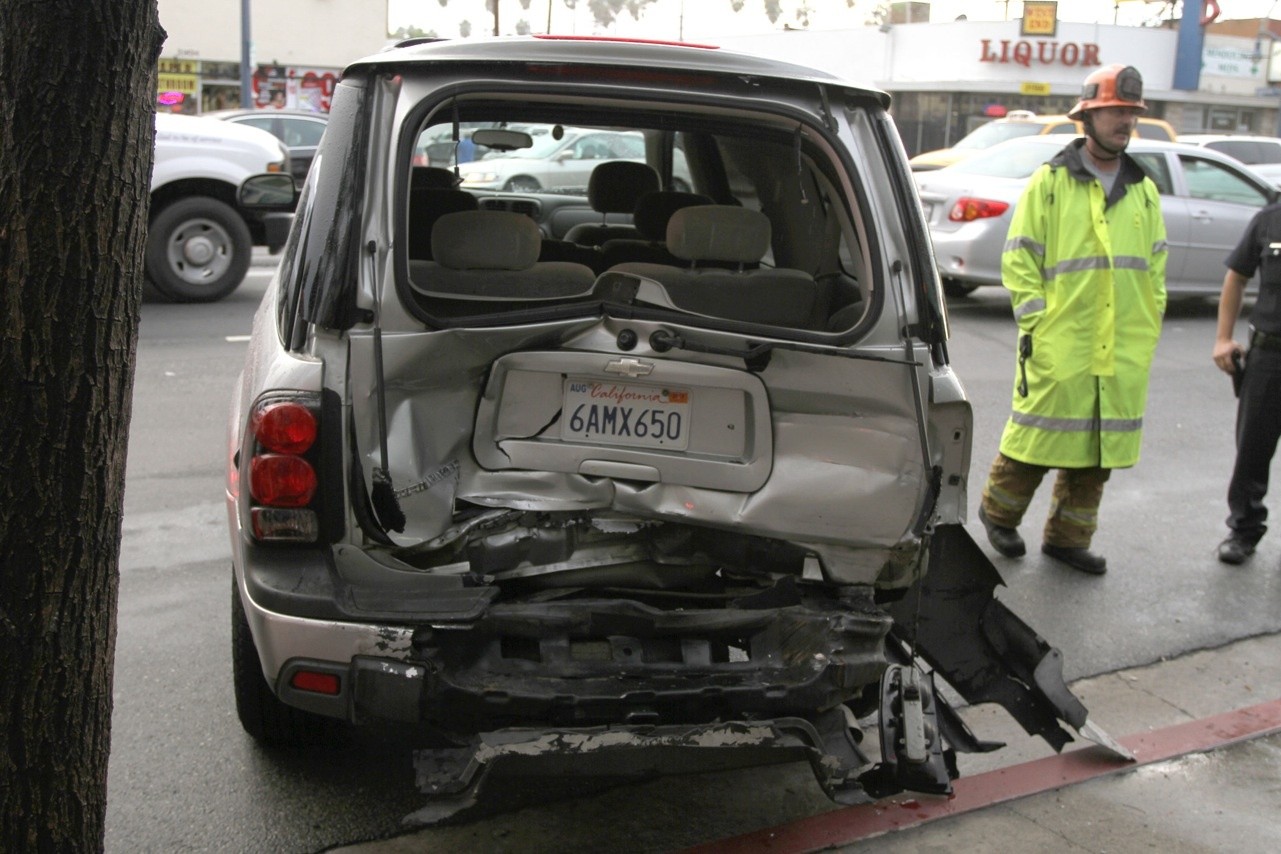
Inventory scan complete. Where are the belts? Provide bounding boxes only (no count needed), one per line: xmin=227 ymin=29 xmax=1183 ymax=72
xmin=1248 ymin=323 xmax=1281 ymax=350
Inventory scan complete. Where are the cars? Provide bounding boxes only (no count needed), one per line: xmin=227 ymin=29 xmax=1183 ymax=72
xmin=414 ymin=123 xmax=608 ymax=168
xmin=144 ymin=110 xmax=295 ymax=304
xmin=447 ymin=128 xmax=697 ymax=197
xmin=225 ymin=35 xmax=1143 ymax=808
xmin=911 ymin=132 xmax=1281 ymax=299
xmin=208 ymin=110 xmax=431 ymax=199
xmin=1176 ymin=134 xmax=1281 ymax=183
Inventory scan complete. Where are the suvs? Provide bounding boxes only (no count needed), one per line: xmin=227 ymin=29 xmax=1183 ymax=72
xmin=910 ymin=109 xmax=1180 ymax=171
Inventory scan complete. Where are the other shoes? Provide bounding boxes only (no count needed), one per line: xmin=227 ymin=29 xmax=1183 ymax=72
xmin=978 ymin=503 xmax=1026 ymax=558
xmin=1041 ymin=540 xmax=1107 ymax=575
xmin=1216 ymin=532 xmax=1256 ymax=566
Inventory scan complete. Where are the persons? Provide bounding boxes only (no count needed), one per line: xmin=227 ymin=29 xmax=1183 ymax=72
xmin=977 ymin=65 xmax=1170 ymax=575
xmin=1213 ymin=199 xmax=1281 ymax=565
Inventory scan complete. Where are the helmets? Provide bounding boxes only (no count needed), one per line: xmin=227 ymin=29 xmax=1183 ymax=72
xmin=1067 ymin=65 xmax=1147 ymax=121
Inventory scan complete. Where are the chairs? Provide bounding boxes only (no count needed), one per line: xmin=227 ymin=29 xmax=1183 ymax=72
xmin=410 ymin=161 xmax=822 ymax=330
